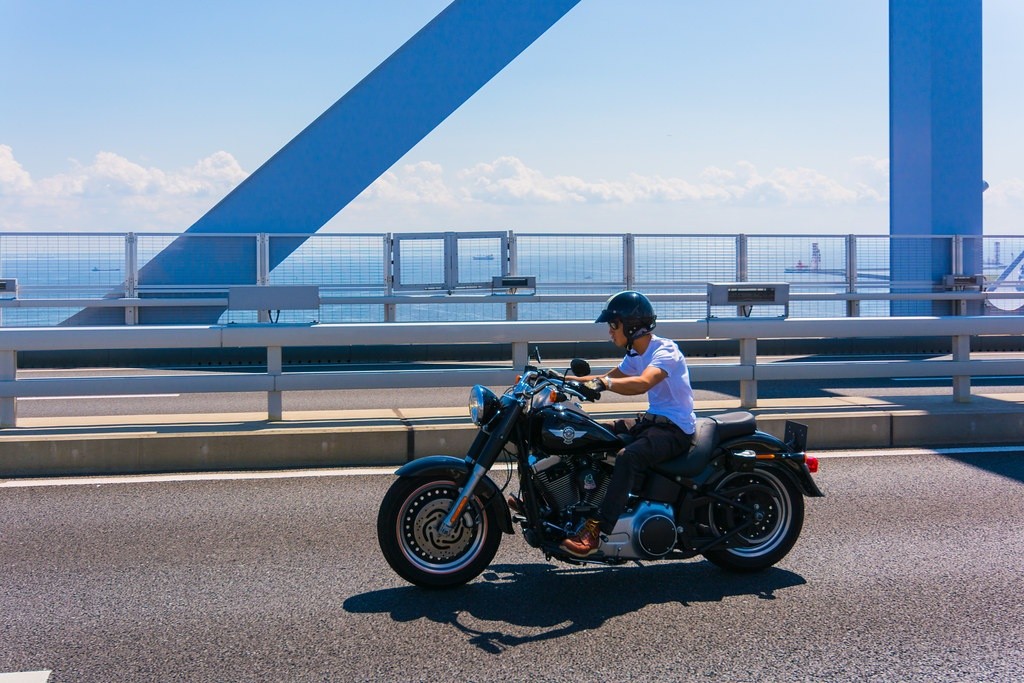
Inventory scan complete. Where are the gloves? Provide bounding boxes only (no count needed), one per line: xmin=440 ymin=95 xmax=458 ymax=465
xmin=579 ymin=377 xmax=606 ymax=402
xmin=546 ymin=368 xmax=564 ymax=381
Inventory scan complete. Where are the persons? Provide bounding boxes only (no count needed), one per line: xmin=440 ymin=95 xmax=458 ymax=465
xmin=540 ymin=292 xmax=697 ymax=559
xmin=809 ymin=242 xmax=821 ymax=270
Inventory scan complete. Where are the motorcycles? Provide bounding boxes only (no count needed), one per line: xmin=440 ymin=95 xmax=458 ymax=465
xmin=377 ymin=346 xmax=826 ymax=590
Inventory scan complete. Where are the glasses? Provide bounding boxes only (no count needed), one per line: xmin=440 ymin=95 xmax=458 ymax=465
xmin=607 ymin=320 xmax=623 ymax=330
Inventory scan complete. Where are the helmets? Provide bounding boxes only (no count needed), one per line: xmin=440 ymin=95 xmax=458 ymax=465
xmin=595 ymin=291 xmax=656 ymax=339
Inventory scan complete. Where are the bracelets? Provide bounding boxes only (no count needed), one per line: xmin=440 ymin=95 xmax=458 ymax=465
xmin=605 ymin=377 xmax=612 ymax=389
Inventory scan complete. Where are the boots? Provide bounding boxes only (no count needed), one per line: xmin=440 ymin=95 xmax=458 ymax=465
xmin=508 ymin=496 xmax=525 ymax=513
xmin=560 ymin=520 xmax=601 ymax=556
